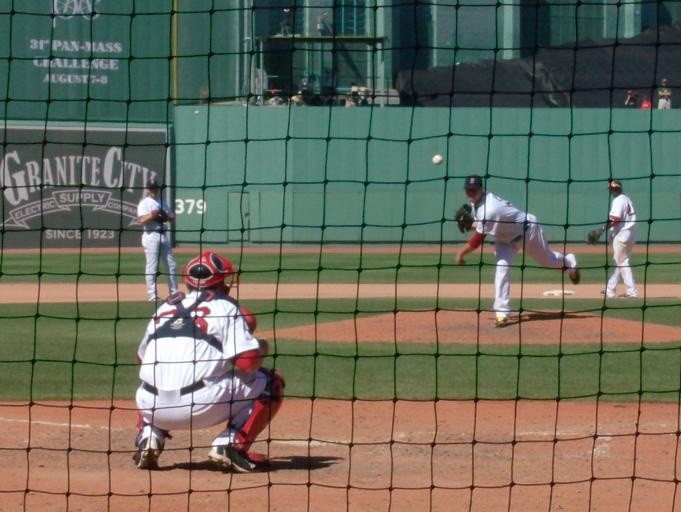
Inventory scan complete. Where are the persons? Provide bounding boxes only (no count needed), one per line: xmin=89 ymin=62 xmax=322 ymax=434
xmin=588 ymin=180 xmax=638 ymax=298
xmin=455 ymin=175 xmax=580 ymax=327
xmin=624 ymin=78 xmax=675 ymax=110
xmin=137 ymin=180 xmax=178 ymax=302
xmin=246 ymin=68 xmax=370 ymax=108
xmin=132 ymin=252 xmax=286 ymax=473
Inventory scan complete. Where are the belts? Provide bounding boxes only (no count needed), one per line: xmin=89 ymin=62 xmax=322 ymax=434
xmin=141 ymin=381 xmax=205 ymax=398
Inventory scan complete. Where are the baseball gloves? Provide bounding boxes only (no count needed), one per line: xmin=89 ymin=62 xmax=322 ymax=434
xmin=456 ymin=205 xmax=475 ymax=233
xmin=588 ymin=228 xmax=604 ymax=244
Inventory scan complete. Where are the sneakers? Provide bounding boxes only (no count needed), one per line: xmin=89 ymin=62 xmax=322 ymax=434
xmin=601 ymin=290 xmax=637 ymax=300
xmin=568 ymin=254 xmax=582 ymax=284
xmin=208 ymin=438 xmax=265 ymax=473
xmin=131 ymin=431 xmax=164 ymax=469
xmin=493 ymin=316 xmax=508 ymax=327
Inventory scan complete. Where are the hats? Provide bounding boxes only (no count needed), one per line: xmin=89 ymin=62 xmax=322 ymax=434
xmin=147 ymin=179 xmax=159 ymax=188
xmin=463 ymin=174 xmax=483 ymax=191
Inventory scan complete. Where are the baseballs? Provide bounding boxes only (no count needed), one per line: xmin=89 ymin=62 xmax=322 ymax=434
xmin=432 ymin=155 xmax=442 ymax=165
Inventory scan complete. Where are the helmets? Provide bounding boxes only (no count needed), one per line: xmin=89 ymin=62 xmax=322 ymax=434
xmin=181 ymin=251 xmax=234 ymax=289
xmin=608 ymin=180 xmax=622 ymax=193
